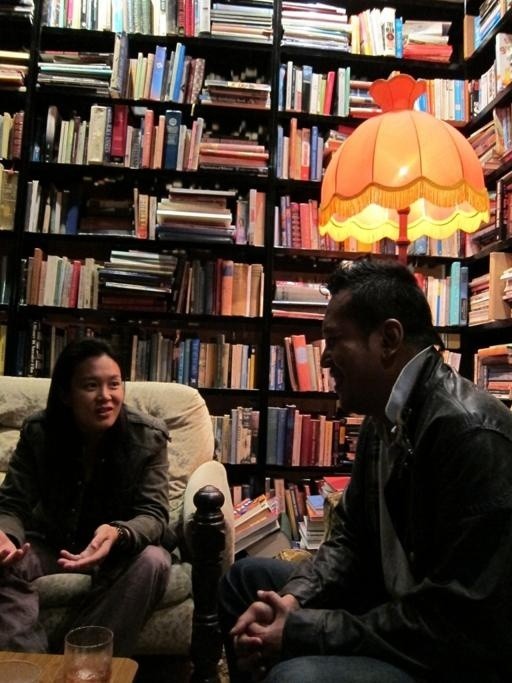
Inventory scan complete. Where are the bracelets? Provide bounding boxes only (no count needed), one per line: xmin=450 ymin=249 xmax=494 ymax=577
xmin=116 ymin=527 xmax=123 ymax=538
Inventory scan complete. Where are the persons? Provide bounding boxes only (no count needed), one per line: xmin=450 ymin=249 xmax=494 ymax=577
xmin=217 ymin=260 xmax=511 ymax=592
xmin=0 ymin=336 xmax=172 ymax=657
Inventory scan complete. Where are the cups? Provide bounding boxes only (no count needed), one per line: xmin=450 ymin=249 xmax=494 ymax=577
xmin=63 ymin=624 xmax=114 ymax=682
xmin=0 ymin=660 xmax=42 ymax=683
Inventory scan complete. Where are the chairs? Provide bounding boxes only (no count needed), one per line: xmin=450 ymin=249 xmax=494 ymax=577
xmin=1 ymin=377 xmax=238 ymax=658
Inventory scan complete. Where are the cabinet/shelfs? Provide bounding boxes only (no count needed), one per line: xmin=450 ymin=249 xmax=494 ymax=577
xmin=0 ymin=0 xmax=512 ymax=562
